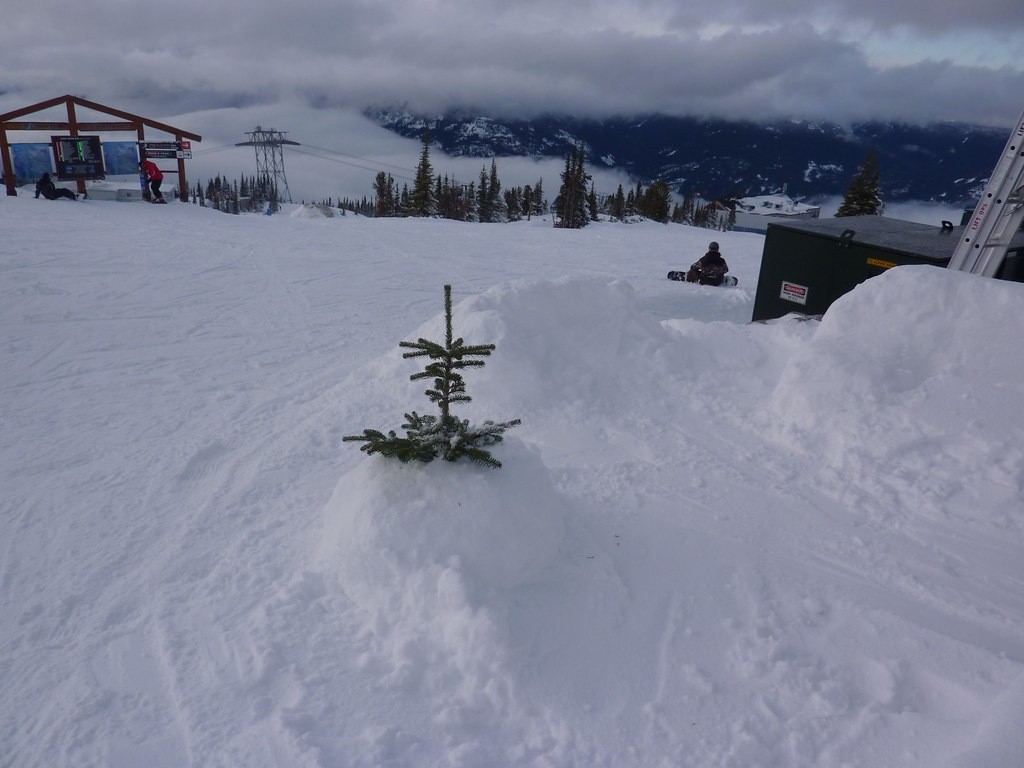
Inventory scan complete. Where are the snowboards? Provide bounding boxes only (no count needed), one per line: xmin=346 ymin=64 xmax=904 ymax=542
xmin=667 ymin=270 xmax=739 ymax=287
xmin=74 ymin=192 xmax=87 ymax=199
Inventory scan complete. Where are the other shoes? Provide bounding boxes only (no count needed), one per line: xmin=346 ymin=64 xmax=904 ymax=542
xmin=678 ymin=274 xmax=688 ymax=281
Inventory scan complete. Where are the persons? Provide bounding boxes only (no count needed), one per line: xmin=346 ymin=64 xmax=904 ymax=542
xmin=139 ymin=161 xmax=163 ymax=203
xmin=34 ymin=172 xmax=79 ymax=200
xmin=687 ymin=242 xmax=729 ymax=286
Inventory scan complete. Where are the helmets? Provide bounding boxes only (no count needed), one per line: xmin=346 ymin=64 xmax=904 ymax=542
xmin=709 ymin=242 xmax=719 ymax=250
